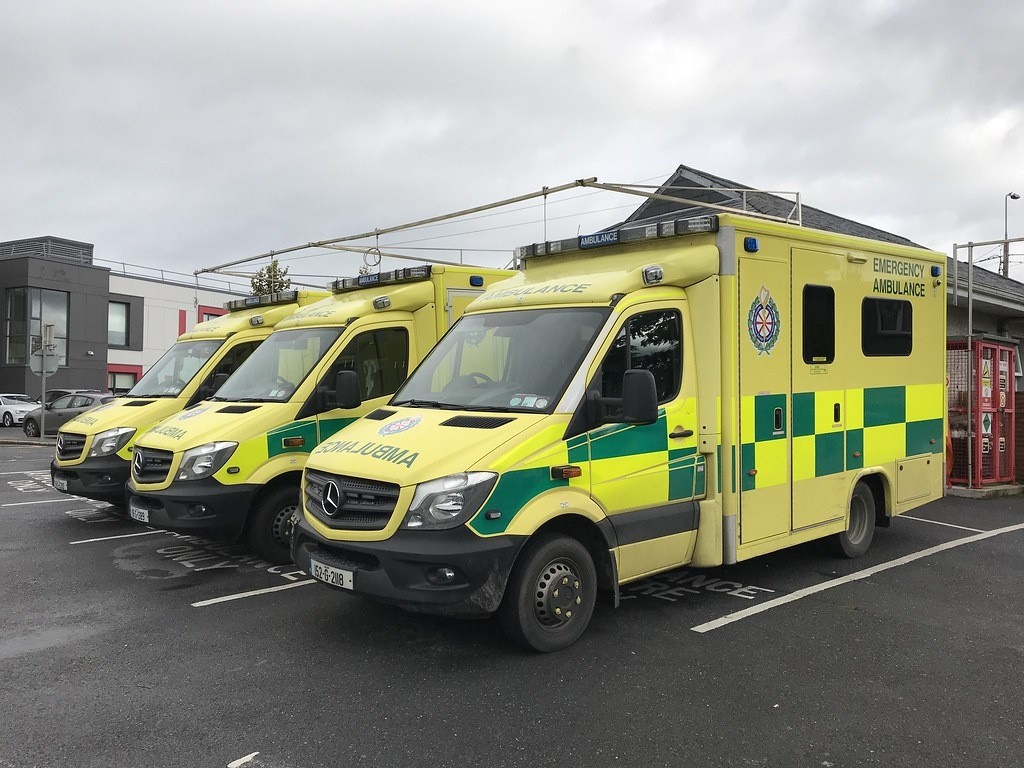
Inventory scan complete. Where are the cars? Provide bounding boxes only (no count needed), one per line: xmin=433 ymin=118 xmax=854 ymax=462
xmin=0 ymin=393 xmax=41 ymax=427
xmin=23 ymin=393 xmax=118 ymax=438
xmin=34 ymin=389 xmax=101 ymax=407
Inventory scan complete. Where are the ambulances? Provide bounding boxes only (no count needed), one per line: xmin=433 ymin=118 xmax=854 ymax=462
xmin=125 ymin=265 xmax=520 ymax=566
xmin=291 ymin=214 xmax=949 ymax=654
xmin=50 ymin=290 xmax=335 ymax=510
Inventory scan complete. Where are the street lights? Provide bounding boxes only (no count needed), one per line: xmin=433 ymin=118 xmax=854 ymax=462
xmin=1002 ymin=192 xmax=1020 ymax=278
